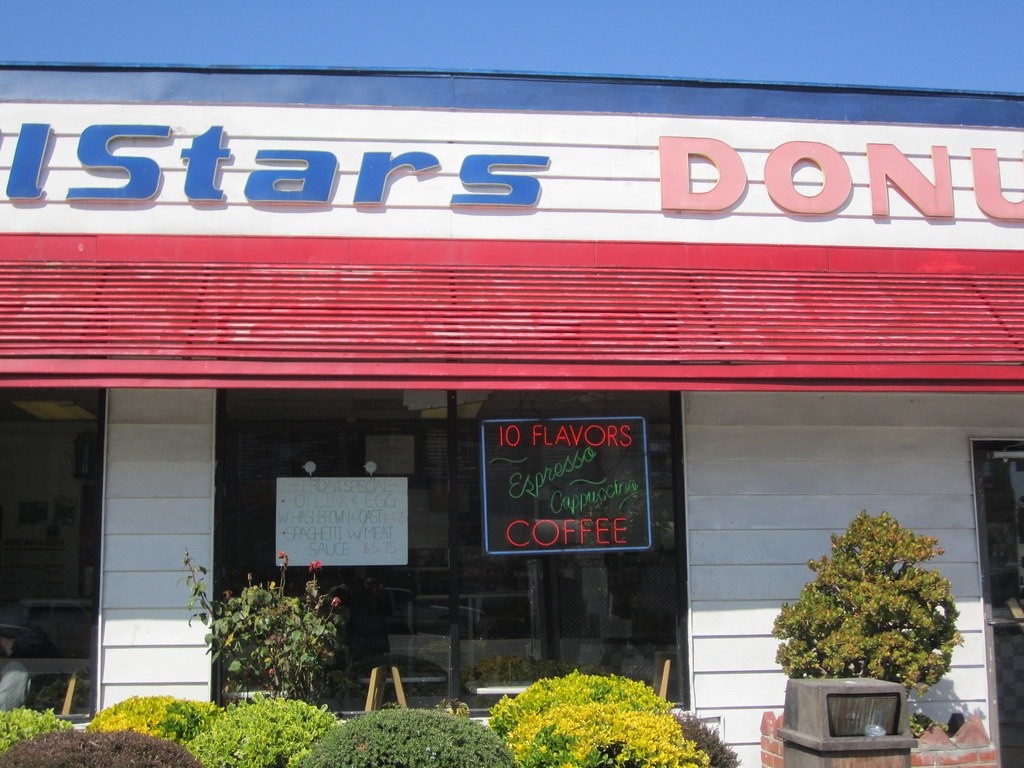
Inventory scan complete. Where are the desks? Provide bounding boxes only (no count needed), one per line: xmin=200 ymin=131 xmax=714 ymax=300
xmin=465 ymin=678 xmax=533 ymax=696
xmin=222 ymin=692 xmax=295 ymax=699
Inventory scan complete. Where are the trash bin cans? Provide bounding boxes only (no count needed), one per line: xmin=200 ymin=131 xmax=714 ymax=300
xmin=777 ymin=677 xmax=918 ymax=768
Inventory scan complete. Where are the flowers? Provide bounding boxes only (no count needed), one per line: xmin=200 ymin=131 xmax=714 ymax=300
xmin=182 ymin=548 xmax=343 ymax=700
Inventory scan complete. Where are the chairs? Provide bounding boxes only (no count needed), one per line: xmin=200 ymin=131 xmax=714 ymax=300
xmin=333 ymin=663 xmax=407 ymax=715
